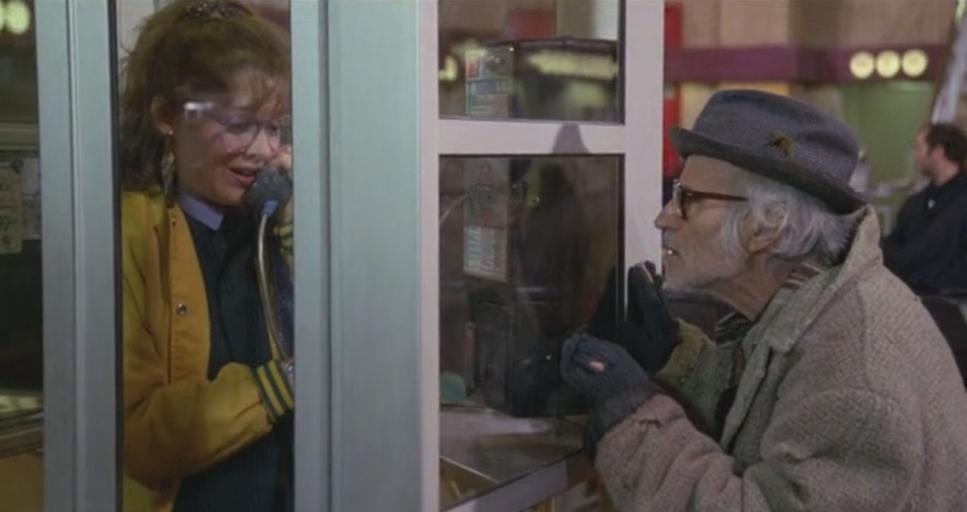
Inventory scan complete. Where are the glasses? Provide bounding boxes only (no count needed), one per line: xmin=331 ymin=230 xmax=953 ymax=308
xmin=672 ymin=182 xmax=749 ymax=224
xmin=204 ymin=107 xmax=292 ymax=153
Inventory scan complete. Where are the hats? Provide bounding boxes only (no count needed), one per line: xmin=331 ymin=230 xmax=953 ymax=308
xmin=667 ymin=89 xmax=866 ymax=215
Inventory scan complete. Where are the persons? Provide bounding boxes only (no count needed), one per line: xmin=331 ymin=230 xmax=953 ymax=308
xmin=882 ymin=116 xmax=966 ymax=372
xmin=121 ymin=0 xmax=293 ymax=510
xmin=566 ymin=87 xmax=965 ymax=509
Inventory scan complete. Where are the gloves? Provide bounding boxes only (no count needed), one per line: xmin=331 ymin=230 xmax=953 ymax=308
xmin=620 ymin=259 xmax=681 ymax=372
xmin=559 ymin=327 xmax=660 ymax=444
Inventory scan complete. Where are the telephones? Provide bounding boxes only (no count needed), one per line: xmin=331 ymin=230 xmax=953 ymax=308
xmin=240 ymin=164 xmax=292 ymax=213
